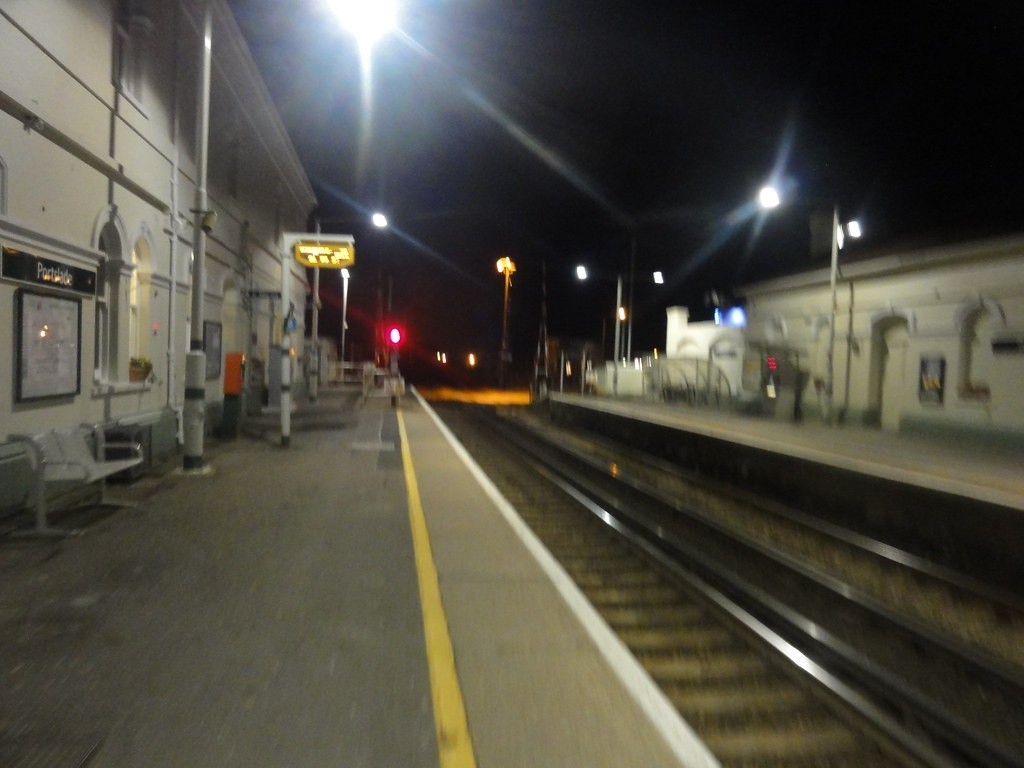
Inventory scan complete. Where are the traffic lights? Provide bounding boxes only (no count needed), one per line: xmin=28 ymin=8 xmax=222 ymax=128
xmin=389 ymin=326 xmax=403 ymax=350
xmin=573 ymin=263 xmax=590 ymax=397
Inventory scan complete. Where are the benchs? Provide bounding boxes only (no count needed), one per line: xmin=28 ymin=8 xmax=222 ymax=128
xmin=4 ymin=422 xmax=146 ymax=539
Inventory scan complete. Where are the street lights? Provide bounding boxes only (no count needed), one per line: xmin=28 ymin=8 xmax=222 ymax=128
xmin=370 ymin=212 xmax=385 ymax=395
xmin=495 ymin=255 xmax=516 ymax=389
xmin=175 ymin=1 xmax=387 ymax=475
xmin=835 ymin=218 xmax=863 ymax=422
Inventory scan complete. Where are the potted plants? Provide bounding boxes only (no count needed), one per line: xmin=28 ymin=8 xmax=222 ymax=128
xmin=129 ymin=356 xmax=163 ymax=387
